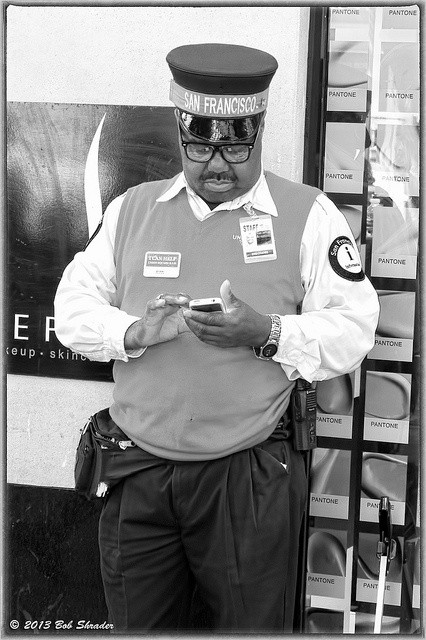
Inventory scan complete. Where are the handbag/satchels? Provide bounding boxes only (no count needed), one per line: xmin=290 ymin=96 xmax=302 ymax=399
xmin=75 ymin=408 xmax=166 ymax=501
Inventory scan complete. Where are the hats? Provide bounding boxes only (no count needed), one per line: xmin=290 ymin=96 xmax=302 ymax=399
xmin=164 ymin=44 xmax=278 ymax=143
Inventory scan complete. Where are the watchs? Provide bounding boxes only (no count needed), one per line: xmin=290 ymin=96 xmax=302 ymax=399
xmin=253 ymin=314 xmax=281 ymax=360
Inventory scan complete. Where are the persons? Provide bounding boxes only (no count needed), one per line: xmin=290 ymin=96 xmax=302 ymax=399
xmin=52 ymin=43 xmax=380 ymax=632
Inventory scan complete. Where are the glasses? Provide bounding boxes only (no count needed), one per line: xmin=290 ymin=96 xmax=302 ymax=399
xmin=179 ymin=130 xmax=259 ymax=164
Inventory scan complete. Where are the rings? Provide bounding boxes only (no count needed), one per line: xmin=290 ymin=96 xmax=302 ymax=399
xmin=156 ymin=294 xmax=167 ymax=308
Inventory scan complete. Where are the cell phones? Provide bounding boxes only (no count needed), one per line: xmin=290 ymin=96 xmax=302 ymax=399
xmin=189 ymin=298 xmax=228 ymax=314
